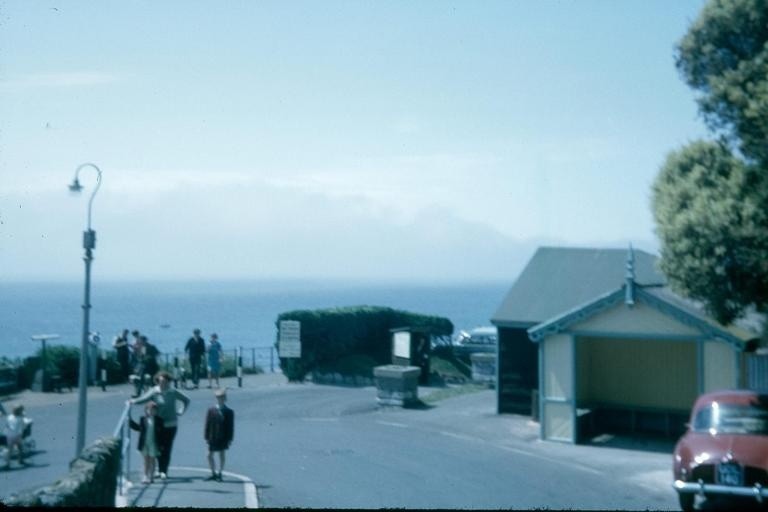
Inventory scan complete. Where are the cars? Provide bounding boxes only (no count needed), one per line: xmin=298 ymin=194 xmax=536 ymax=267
xmin=667 ymin=384 xmax=767 ymax=511
xmin=454 ymin=326 xmax=504 ymax=353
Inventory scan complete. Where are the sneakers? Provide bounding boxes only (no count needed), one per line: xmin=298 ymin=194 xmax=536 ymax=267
xmin=139 ymin=471 xmax=167 ymax=484
xmin=191 ymin=384 xmax=220 ymax=389
xmin=206 ymin=474 xmax=224 ymax=483
xmin=6 ymin=459 xmax=28 ymax=467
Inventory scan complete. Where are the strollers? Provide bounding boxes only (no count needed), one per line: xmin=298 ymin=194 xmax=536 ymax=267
xmin=0 ymin=410 xmax=37 ymax=457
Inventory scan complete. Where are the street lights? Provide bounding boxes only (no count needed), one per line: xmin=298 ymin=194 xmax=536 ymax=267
xmin=69 ymin=159 xmax=104 ymax=465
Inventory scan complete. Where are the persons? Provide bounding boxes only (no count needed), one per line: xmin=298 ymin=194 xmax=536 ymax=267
xmin=112 ymin=327 xmax=130 ymax=378
xmin=127 ymin=330 xmax=143 ymax=376
xmin=131 ymin=335 xmax=162 ymax=399
xmin=207 ymin=334 xmax=221 ymax=388
xmin=1 ymin=404 xmax=29 ymax=469
xmin=129 ymin=401 xmax=166 ymax=482
xmin=204 ymin=388 xmax=234 ymax=481
xmin=129 ymin=372 xmax=192 ymax=479
xmin=183 ymin=328 xmax=205 ymax=388
